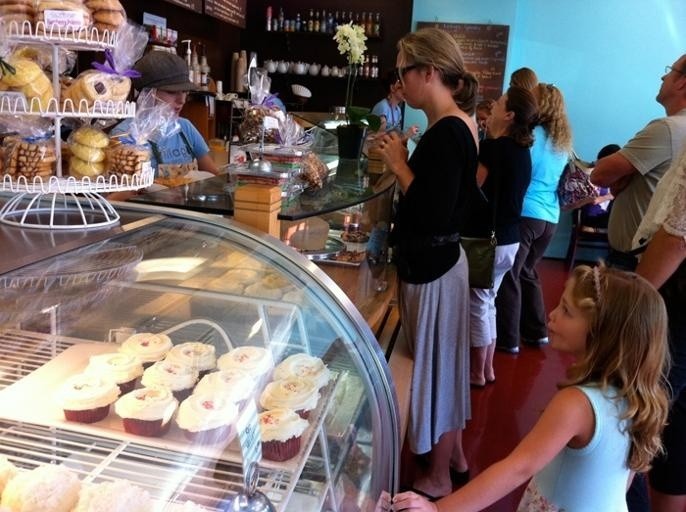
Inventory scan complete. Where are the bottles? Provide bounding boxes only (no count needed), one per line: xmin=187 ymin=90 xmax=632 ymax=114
xmin=334 ymin=10 xmax=339 ymax=27
xmin=341 ymin=11 xmax=346 ymax=24
xmin=265 ymin=6 xmax=272 ymax=31
xmin=290 ymin=19 xmax=295 ymax=32
xmin=360 ymin=12 xmax=366 ymax=30
xmin=284 ymin=19 xmax=290 ymax=32
xmin=272 ymin=18 xmax=278 ymax=31
xmin=191 ymin=44 xmax=201 ymax=86
xmin=199 ymin=43 xmax=209 ymax=86
xmin=355 ymin=13 xmax=359 ymax=25
xmin=320 ymin=10 xmax=326 ymax=32
xmin=358 ymin=54 xmax=379 ymax=80
xmin=348 ymin=11 xmax=353 ymax=23
xmin=302 ymin=20 xmax=308 ymax=31
xmin=374 ymin=12 xmax=381 ymax=38
xmin=326 ymin=12 xmax=334 ymax=33
xmin=296 ymin=12 xmax=301 ymax=30
xmin=278 ymin=7 xmax=284 ymax=31
xmin=366 ymin=12 xmax=374 ymax=37
xmin=308 ymin=8 xmax=313 ymax=32
xmin=314 ymin=9 xmax=320 ymax=31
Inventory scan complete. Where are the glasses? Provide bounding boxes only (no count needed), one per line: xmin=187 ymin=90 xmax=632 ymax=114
xmin=396 ymin=63 xmax=420 ymax=80
xmin=664 ymin=65 xmax=685 ymax=77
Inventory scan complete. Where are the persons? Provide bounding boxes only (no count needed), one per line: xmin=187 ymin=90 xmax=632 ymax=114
xmin=468 ymin=86 xmax=539 ymax=388
xmin=106 ymin=50 xmax=221 ymax=201
xmin=375 ymin=28 xmax=480 ymax=504
xmin=368 ymin=70 xmax=420 ymax=145
xmin=475 ymin=98 xmax=497 ymax=139
xmin=510 ymin=67 xmax=539 ymax=92
xmin=629 ymin=146 xmax=686 ymax=512
xmin=580 ymin=144 xmax=621 ymax=229
xmin=495 ymin=82 xmax=572 ymax=354
xmin=590 ymin=54 xmax=686 ymax=271
xmin=390 ymin=267 xmax=671 ymax=512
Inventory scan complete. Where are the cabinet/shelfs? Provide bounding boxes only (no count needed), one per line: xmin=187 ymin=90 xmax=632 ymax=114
xmin=0 ymin=192 xmax=401 ymax=512
xmin=251 ymin=0 xmax=415 ymax=112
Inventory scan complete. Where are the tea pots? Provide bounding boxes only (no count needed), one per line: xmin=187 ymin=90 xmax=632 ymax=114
xmin=278 ymin=60 xmax=289 ymax=72
xmin=308 ymin=61 xmax=320 ymax=75
xmin=291 ymin=60 xmax=308 ymax=75
xmin=263 ymin=59 xmax=278 ymax=72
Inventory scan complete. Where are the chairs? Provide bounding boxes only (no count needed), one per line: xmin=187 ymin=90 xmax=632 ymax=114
xmin=565 ymin=205 xmax=609 ymax=274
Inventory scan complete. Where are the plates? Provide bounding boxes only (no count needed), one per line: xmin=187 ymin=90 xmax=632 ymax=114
xmin=0 ymin=344 xmax=337 ymax=472
xmin=315 ymin=229 xmax=371 ymax=266
xmin=302 ymin=236 xmax=347 ymax=259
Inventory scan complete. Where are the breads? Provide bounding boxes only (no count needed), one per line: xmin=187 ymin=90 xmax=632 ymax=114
xmin=69 ymin=142 xmax=105 ymax=163
xmin=0 ymin=0 xmax=92 ymax=39
xmin=28 ymin=89 xmax=55 ymax=113
xmin=11 ymin=72 xmax=49 ymax=99
xmin=70 ymin=156 xmax=104 ymax=176
xmin=85 ymin=0 xmax=124 ymax=11
xmin=91 ymin=21 xmax=119 ymax=35
xmin=73 ymin=126 xmax=110 ymax=148
xmin=2 ymin=60 xmax=41 ymax=87
xmin=92 ymin=10 xmax=125 ymax=25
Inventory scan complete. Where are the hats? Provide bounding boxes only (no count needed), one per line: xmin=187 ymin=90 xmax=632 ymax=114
xmin=131 ymin=50 xmax=199 ymax=93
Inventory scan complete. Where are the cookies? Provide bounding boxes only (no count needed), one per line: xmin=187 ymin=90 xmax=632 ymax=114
xmin=106 ymin=143 xmax=151 ymax=184
xmin=0 ymin=136 xmax=56 ymax=183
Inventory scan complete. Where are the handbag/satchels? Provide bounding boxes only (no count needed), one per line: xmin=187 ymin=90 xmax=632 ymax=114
xmin=461 ymin=185 xmax=499 ymax=290
xmin=557 ymin=149 xmax=600 ymax=212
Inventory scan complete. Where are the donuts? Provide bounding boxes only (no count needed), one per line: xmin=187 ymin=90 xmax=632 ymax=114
xmin=65 ymin=72 xmax=132 ymax=112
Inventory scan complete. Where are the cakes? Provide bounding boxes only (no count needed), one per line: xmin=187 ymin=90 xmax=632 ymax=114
xmin=85 ymin=352 xmax=145 ymax=397
xmin=113 ymin=387 xmax=180 ymax=437
xmin=193 ymin=368 xmax=254 ymax=412
xmin=217 ymin=345 xmax=273 ymax=379
xmin=120 ymin=332 xmax=173 ymax=371
xmin=257 ymin=408 xmax=309 ymax=462
xmin=176 ymin=392 xmax=238 ymax=445
xmin=165 ymin=341 xmax=218 ymax=377
xmin=59 ymin=372 xmax=122 ymax=424
xmin=273 ymin=353 xmax=331 ymax=391
xmin=259 ymin=377 xmax=323 ymax=420
xmin=141 ymin=360 xmax=199 ymax=401
